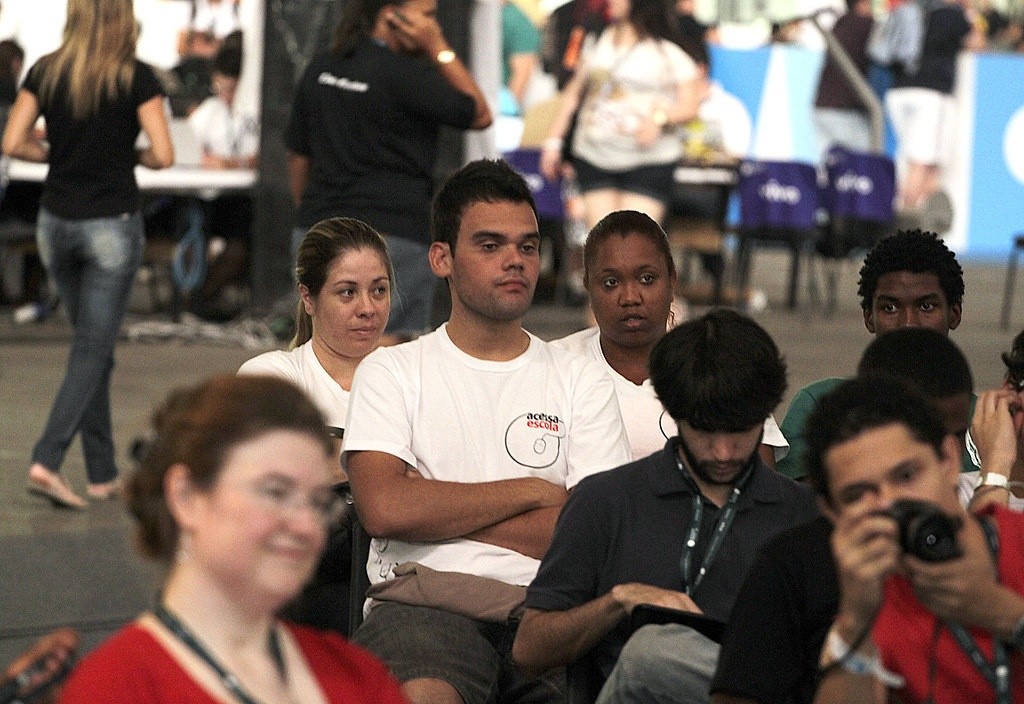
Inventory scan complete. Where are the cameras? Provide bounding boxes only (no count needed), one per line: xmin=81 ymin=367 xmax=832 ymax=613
xmin=874 ymin=497 xmax=1000 ymax=561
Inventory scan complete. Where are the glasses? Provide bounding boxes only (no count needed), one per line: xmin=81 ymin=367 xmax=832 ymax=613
xmin=216 ymin=474 xmax=345 ymax=521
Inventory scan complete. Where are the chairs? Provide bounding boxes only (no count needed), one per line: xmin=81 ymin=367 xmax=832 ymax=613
xmin=495 ymin=143 xmax=900 ymax=317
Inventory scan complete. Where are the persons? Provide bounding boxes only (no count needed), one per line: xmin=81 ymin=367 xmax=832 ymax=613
xmin=534 ymin=0 xmax=710 ymax=237
xmin=235 ymin=216 xmax=392 ymax=639
xmin=805 ymin=374 xmax=1024 ymax=704
xmin=951 ymin=331 xmax=1024 ymax=515
xmin=0 ymin=627 xmax=79 ymax=703
xmin=545 ymin=211 xmax=791 ymax=474
xmin=3 ymin=0 xmax=176 ymax=511
xmin=281 ymin=1 xmax=494 ymax=336
xmin=341 ymin=160 xmax=635 ymax=704
xmin=58 ymin=372 xmax=413 ymax=704
xmin=0 ymin=0 xmax=1024 ymax=323
xmin=513 ymin=309 xmax=840 ymax=704
xmin=774 ymin=228 xmax=982 ymax=511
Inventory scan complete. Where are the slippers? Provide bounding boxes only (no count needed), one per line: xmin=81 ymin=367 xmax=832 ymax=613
xmin=25 ymin=470 xmax=89 ymax=512
xmin=87 ymin=477 xmax=128 ymax=500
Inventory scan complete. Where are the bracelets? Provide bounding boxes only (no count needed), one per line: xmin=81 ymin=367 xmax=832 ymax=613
xmin=973 ymin=471 xmax=1010 ymax=491
xmin=652 ymin=108 xmax=670 ymax=131
xmin=541 ymin=138 xmax=565 ymax=153
xmin=824 ymin=627 xmax=905 ymax=689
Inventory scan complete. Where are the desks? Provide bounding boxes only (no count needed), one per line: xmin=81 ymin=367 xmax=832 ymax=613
xmin=1 ymin=156 xmax=739 ymax=326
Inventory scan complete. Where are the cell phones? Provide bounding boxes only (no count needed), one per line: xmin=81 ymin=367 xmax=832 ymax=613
xmin=628 ymin=603 xmax=726 ymax=646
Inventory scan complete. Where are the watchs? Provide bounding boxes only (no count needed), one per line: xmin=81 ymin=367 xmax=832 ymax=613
xmin=432 ymin=50 xmax=457 ymax=66
